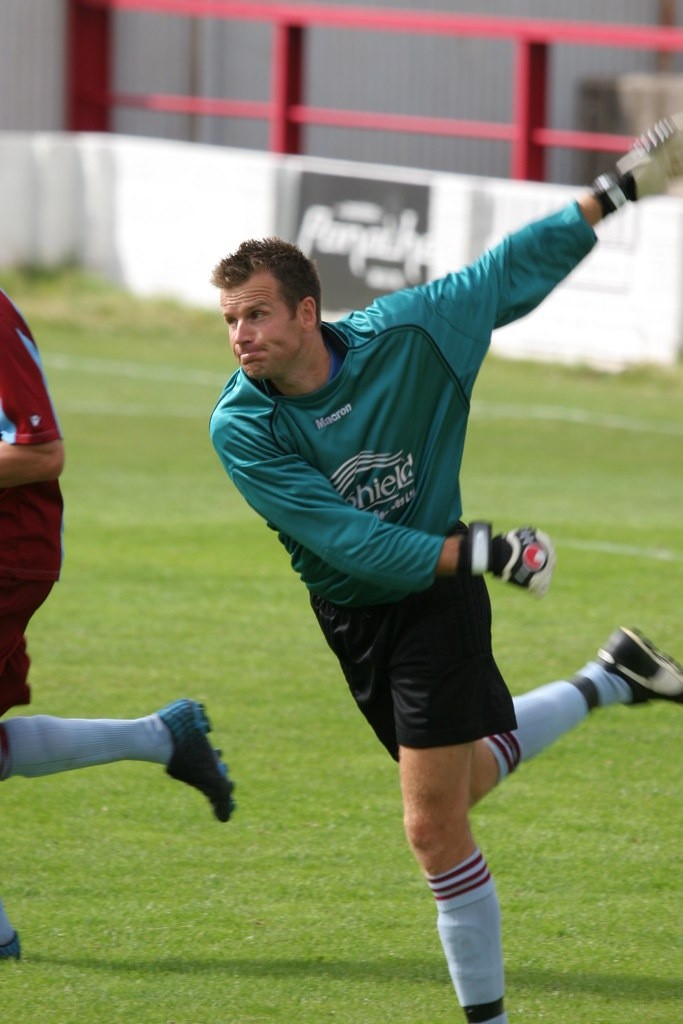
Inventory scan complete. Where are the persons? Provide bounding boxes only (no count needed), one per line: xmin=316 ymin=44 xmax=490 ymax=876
xmin=0 ymin=291 xmax=236 ymax=960
xmin=208 ymin=131 xmax=683 ymax=1024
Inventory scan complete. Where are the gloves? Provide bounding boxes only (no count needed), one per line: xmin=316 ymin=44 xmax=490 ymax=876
xmin=469 ymin=519 xmax=553 ymax=598
xmin=594 ymin=118 xmax=682 ymax=212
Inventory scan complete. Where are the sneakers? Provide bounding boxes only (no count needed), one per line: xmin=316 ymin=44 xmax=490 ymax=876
xmin=157 ymin=699 xmax=236 ymax=824
xmin=596 ymin=626 xmax=683 ymax=706
